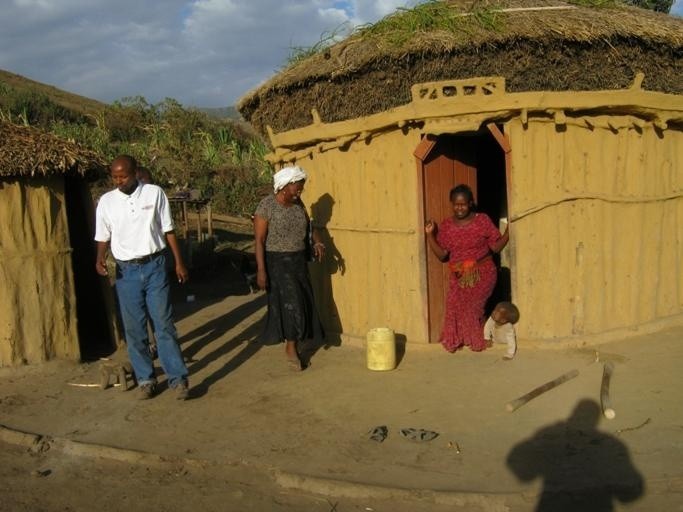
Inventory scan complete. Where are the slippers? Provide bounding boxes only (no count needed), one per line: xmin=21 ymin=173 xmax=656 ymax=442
xmin=288 ymin=360 xmax=303 ymax=372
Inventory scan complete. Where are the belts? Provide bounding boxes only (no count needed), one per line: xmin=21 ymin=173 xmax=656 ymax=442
xmin=123 ymin=251 xmax=160 ymax=265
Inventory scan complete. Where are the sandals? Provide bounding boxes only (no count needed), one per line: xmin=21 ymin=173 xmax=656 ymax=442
xmin=399 ymin=428 xmax=437 ymax=441
xmin=368 ymin=426 xmax=389 ymax=441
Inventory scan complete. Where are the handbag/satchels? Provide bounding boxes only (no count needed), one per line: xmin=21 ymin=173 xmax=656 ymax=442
xmin=306 ymin=221 xmax=316 ymax=262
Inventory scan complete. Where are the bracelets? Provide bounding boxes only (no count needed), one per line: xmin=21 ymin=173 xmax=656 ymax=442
xmin=312 ymin=242 xmax=326 ymax=249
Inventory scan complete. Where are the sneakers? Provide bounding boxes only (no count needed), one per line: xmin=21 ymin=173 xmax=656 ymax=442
xmin=174 ymin=383 xmax=190 ymax=400
xmin=136 ymin=382 xmax=156 ymax=400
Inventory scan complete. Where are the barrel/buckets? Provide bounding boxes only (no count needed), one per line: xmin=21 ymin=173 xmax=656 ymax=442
xmin=366 ymin=327 xmax=396 ymax=371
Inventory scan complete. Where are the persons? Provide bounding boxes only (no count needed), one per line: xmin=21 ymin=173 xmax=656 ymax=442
xmin=483 ymin=301 xmax=520 ymax=360
xmin=93 ymin=153 xmax=193 ymax=401
xmin=423 ymin=181 xmax=508 ymax=354
xmin=252 ymin=164 xmax=328 ymax=372
xmin=134 ymin=165 xmax=160 ymax=360
